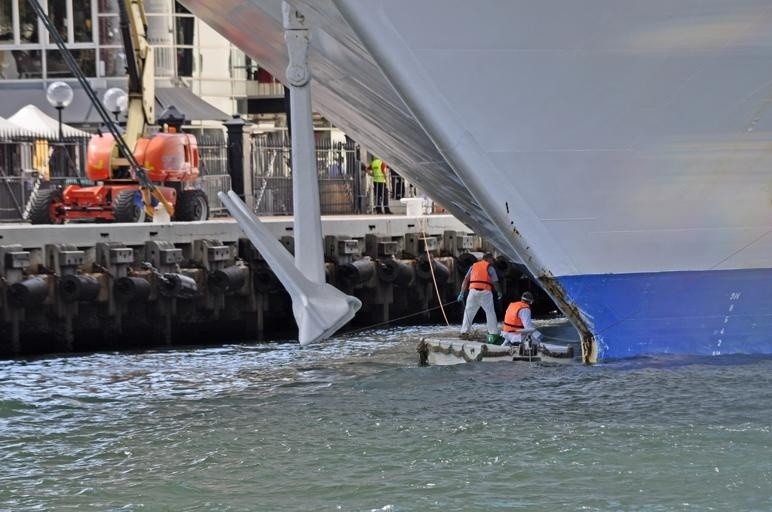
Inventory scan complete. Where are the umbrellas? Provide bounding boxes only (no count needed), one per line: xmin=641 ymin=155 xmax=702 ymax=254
xmin=0 ymin=115 xmax=44 ymax=142
xmin=5 ymin=103 xmax=96 ymax=139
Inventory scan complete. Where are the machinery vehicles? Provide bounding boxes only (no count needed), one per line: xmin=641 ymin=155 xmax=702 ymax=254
xmin=30 ymin=0 xmax=211 ymax=224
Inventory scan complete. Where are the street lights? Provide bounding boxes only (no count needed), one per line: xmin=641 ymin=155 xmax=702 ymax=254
xmin=103 ymin=87 xmax=127 ymax=122
xmin=45 ymin=81 xmax=73 ymax=142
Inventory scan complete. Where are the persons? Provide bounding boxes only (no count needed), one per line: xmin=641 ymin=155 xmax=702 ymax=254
xmin=365 ymin=157 xmax=394 ymax=215
xmin=501 ymin=290 xmax=572 ymax=354
xmin=457 ymin=252 xmax=503 ymax=340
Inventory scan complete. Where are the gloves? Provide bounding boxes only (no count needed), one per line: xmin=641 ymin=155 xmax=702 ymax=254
xmin=457 ymin=292 xmax=465 ymax=303
xmin=497 ymin=292 xmax=503 ymax=300
xmin=539 ymin=335 xmax=546 ymax=342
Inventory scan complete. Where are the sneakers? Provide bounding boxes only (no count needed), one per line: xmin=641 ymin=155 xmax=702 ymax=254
xmin=376 ymin=209 xmax=393 ymax=214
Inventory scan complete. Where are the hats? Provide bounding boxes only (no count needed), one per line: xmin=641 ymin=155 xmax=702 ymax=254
xmin=483 ymin=253 xmax=497 ymax=263
xmin=521 ymin=291 xmax=535 ymax=305
